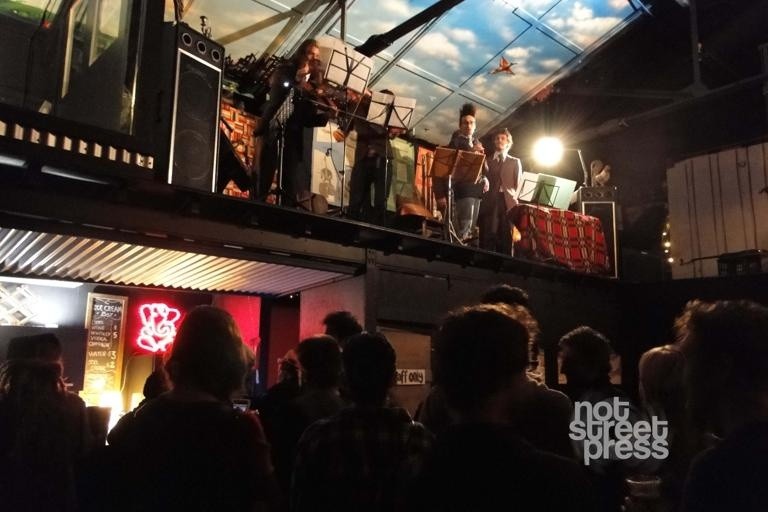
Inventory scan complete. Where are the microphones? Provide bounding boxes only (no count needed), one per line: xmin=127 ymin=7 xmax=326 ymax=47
xmin=473 ymin=138 xmax=485 ymax=154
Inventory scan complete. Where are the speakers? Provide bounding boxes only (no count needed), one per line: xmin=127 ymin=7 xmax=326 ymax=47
xmin=132 ymin=20 xmax=226 ymax=194
xmin=575 ymin=187 xmax=623 ymax=281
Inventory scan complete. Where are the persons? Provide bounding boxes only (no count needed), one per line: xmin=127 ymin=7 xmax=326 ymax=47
xmin=246 ymin=38 xmax=321 ymax=205
xmin=334 ymin=85 xmax=394 ymax=216
xmin=433 ymin=102 xmax=490 ymax=248
xmin=0 ymin=282 xmax=767 ymax=511
xmin=475 ymin=128 xmax=522 ymax=257
xmin=566 ymin=150 xmax=622 ymax=275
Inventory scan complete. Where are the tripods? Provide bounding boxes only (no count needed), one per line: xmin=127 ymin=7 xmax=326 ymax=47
xmin=435 ymin=175 xmax=464 ymax=246
xmin=252 ymin=123 xmax=310 ymax=213
xmin=317 ymin=127 xmax=355 ymax=219
